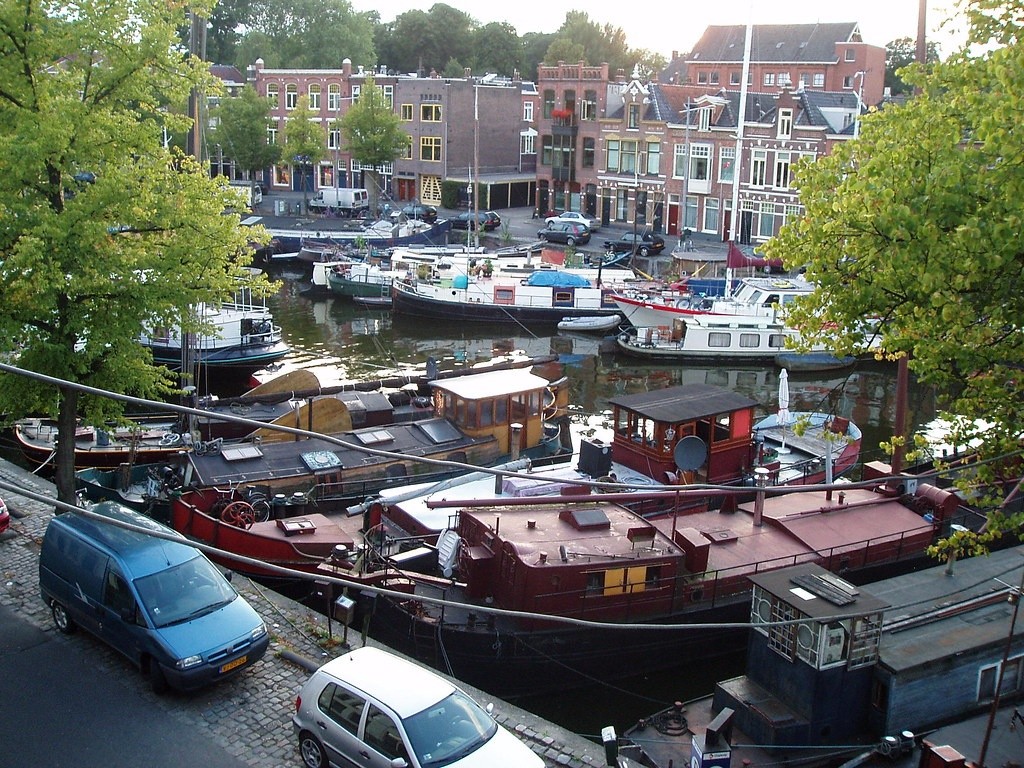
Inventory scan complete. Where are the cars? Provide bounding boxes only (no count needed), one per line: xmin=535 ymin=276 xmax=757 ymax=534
xmin=741 ymin=243 xmax=784 ymax=274
xmin=604 ymin=231 xmax=666 ymax=256
xmin=535 ymin=220 xmax=591 ymax=246
xmin=451 ymin=207 xmax=502 ymax=231
xmin=398 ymin=202 xmax=438 ymax=225
xmin=547 ymin=211 xmax=602 ymax=231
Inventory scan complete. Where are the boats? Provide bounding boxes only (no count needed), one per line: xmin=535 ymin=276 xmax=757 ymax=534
xmin=556 ymin=316 xmax=622 ymax=332
xmin=75 ymin=289 xmax=290 ymax=389
xmin=9 ymin=360 xmax=1024 ymax=768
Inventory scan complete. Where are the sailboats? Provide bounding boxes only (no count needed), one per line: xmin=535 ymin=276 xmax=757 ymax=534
xmin=185 ymin=0 xmax=902 ymax=368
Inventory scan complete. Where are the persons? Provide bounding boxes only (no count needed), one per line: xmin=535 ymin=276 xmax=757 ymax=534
xmin=532 ymin=208 xmax=555 ymax=219
xmin=677 ymin=227 xmax=684 ymax=243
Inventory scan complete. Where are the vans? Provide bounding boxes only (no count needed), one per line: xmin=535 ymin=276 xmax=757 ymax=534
xmin=37 ymin=499 xmax=270 ymax=691
xmin=218 ymin=179 xmax=267 ymax=210
xmin=308 ymin=187 xmax=369 ymax=214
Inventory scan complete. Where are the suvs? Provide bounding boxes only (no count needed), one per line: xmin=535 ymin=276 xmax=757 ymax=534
xmin=290 ymin=646 xmax=546 ymax=767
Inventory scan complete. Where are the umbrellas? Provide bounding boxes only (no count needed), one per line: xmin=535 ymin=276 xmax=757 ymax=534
xmin=777 ymin=368 xmax=791 ymax=451
